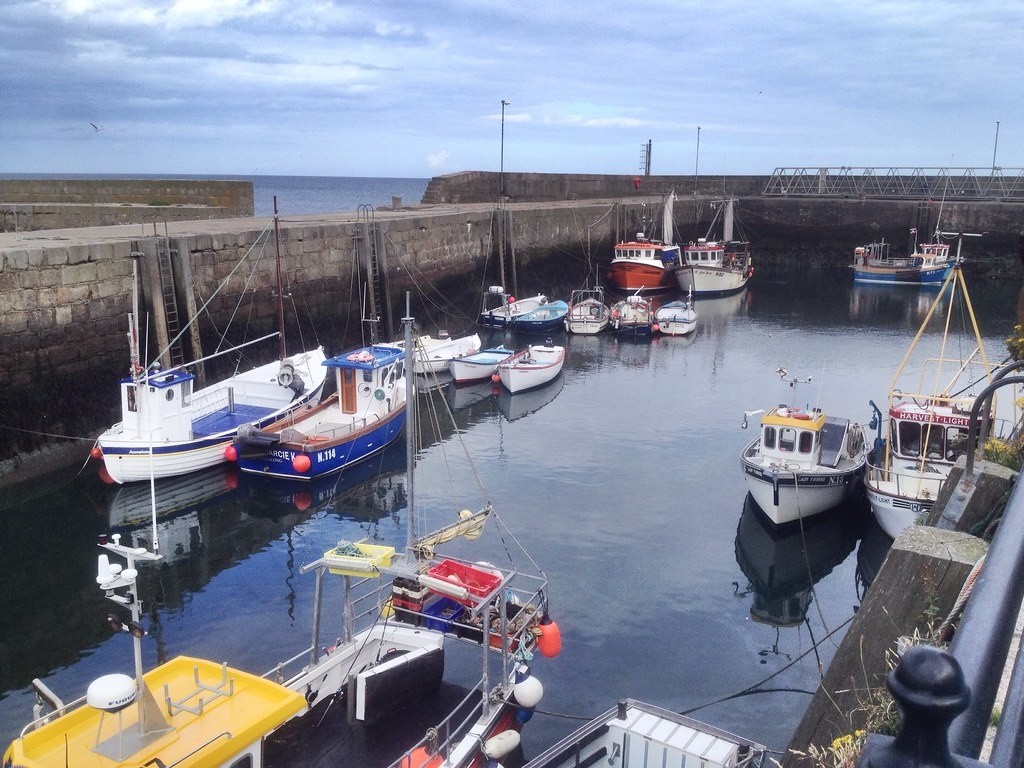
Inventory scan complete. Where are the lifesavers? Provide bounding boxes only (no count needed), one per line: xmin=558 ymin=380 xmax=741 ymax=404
xmin=471 ymin=560 xmax=506 ymax=582
xmin=743 ymin=267 xmax=749 ymax=278
xmin=347 ymin=353 xmax=373 ymax=362
xmin=918 ymin=257 xmax=923 ymax=263
xmin=778 ymin=407 xmax=815 ymax=420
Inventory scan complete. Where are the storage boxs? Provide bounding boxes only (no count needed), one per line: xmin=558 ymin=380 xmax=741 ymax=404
xmin=422 ymin=598 xmax=463 ymax=633
xmin=322 ymin=543 xmax=396 ymax=578
xmin=490 ymin=631 xmax=521 ymax=651
xmin=427 ymin=558 xmax=502 ymax=607
xmin=392 ymin=576 xmax=440 ymax=626
xmin=455 ymin=612 xmax=498 ymax=645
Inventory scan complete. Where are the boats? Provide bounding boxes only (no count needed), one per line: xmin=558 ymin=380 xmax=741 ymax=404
xmin=731 ymin=485 xmax=866 ymax=665
xmin=736 ymin=358 xmax=871 ymax=527
xmin=847 ymin=173 xmax=967 ymax=287
xmin=860 ymin=228 xmax=1019 ymax=547
xmin=480 ymin=292 xmax=549 ymax=330
xmin=494 ymin=370 xmax=565 ymax=423
xmin=445 ymin=343 xmax=515 ymax=385
xmin=654 ymin=284 xmax=699 ymax=336
xmin=2 ymin=294 xmax=566 ymax=767
xmin=673 ymin=196 xmax=755 ymax=297
xmin=608 ymin=231 xmax=678 ymax=297
xmin=402 ymin=331 xmax=482 ymax=375
xmin=495 ymin=336 xmax=566 ymax=394
xmin=513 ymin=299 xmax=569 ymax=333
xmin=101 ymin=458 xmax=244 ymax=535
xmin=608 ymin=284 xmax=653 ymax=333
xmin=521 ymin=695 xmax=769 ymax=768
xmin=562 ymin=261 xmax=610 ymax=336
xmin=371 ymin=334 xmax=453 ymax=359
xmin=223 ymin=341 xmax=415 ymax=483
xmin=445 ymin=378 xmax=496 ymax=413
xmin=83 ymin=192 xmax=329 ymax=487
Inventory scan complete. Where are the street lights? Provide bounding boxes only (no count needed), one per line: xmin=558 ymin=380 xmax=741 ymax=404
xmin=498 ymin=99 xmax=510 ymax=203
xmin=692 ymin=125 xmax=702 ymax=195
xmin=988 ymin=120 xmax=1001 ymax=197
